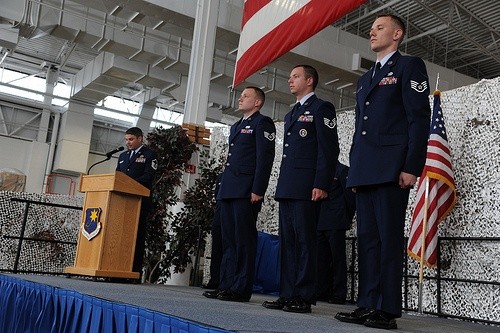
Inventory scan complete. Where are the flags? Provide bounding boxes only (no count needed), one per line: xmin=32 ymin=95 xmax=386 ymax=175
xmin=230 ymin=0 xmax=370 ymax=91
xmin=406 ymin=91 xmax=455 ymax=269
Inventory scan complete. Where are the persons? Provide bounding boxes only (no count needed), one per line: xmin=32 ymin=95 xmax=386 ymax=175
xmin=105 ymin=127 xmax=159 ymax=285
xmin=316 ymin=146 xmax=356 ymax=304
xmin=203 ymin=86 xmax=276 ymax=302
xmin=264 ymin=64 xmax=339 ymax=313
xmin=334 ymin=13 xmax=432 ymax=329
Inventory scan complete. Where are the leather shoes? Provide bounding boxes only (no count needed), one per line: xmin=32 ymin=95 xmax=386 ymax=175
xmin=282 ymin=301 xmax=312 ymax=314
xmin=217 ymin=290 xmax=249 ymax=302
xmin=201 ymin=288 xmax=224 ymax=298
xmin=334 ymin=306 xmax=375 ymax=324
xmin=262 ymin=297 xmax=292 ymax=310
xmin=364 ymin=311 xmax=398 ymax=330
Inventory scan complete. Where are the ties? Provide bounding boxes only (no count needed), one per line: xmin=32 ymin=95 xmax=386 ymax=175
xmin=372 ymin=62 xmax=381 ymax=78
xmin=291 ymin=102 xmax=300 ymax=119
xmin=129 ymin=150 xmax=136 ymax=161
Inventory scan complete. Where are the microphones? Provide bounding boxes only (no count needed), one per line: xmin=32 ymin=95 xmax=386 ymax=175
xmin=106 ymin=146 xmax=124 ymax=156
